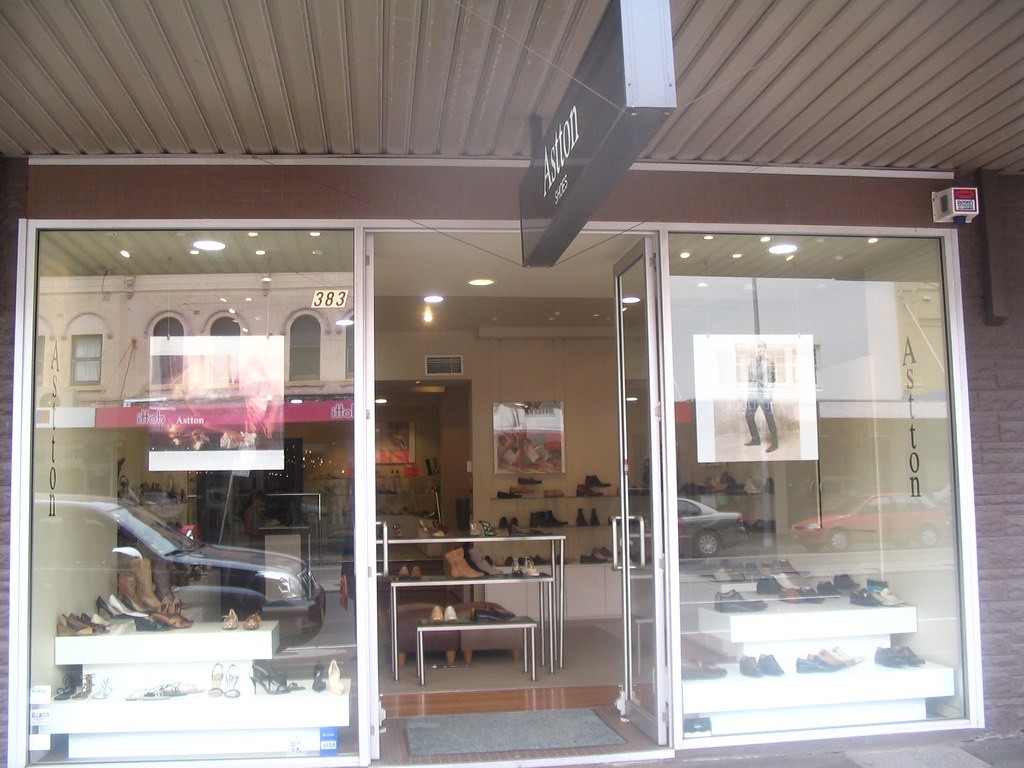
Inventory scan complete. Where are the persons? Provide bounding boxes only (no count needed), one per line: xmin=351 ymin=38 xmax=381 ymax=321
xmin=499 ymin=435 xmax=561 ymax=473
xmin=744 ymin=341 xmax=779 ymax=452
xmin=494 ymin=401 xmax=529 ymax=472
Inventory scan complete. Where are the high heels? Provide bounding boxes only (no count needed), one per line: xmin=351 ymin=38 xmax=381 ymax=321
xmin=393 ymin=519 xmax=539 ymax=538
xmin=54 ymin=660 xmax=345 ymax=699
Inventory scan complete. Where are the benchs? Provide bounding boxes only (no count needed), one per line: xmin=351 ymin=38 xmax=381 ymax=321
xmin=453 ymin=601 xmax=523 ymax=661
xmin=392 ymin=607 xmax=460 ymax=666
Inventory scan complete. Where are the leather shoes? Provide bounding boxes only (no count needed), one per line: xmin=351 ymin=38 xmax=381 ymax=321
xmin=682 ymin=646 xmax=925 ymax=679
xmin=431 ymin=604 xmax=515 ymax=623
xmin=715 ymin=560 xmax=906 ymax=613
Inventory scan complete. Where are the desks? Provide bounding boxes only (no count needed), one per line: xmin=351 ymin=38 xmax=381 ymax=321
xmin=377 ymin=533 xmax=567 ymax=688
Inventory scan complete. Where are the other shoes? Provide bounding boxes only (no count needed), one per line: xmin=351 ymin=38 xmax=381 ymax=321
xmin=765 ymin=445 xmax=779 ymax=453
xmin=745 ymin=439 xmax=760 ymax=447
xmin=55 ymin=556 xmax=261 ymax=636
xmin=499 ymin=508 xmax=600 ymax=528
xmin=496 ymin=472 xmax=764 ymax=498
xmin=397 ymin=545 xmax=622 ymax=582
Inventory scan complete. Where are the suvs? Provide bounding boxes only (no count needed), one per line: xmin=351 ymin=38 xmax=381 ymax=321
xmin=31 ymin=493 xmax=327 ymax=673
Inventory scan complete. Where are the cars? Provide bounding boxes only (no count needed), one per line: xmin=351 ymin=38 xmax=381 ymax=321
xmin=790 ymin=492 xmax=953 ymax=553
xmin=630 ymin=498 xmax=748 ymax=562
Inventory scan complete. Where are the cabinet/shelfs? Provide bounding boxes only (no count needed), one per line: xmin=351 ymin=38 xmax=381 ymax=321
xmin=485 ymin=487 xmax=955 ymax=736
xmin=41 ymin=469 xmax=442 ymax=734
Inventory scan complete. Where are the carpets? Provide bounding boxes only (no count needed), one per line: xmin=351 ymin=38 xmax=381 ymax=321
xmin=401 ymin=707 xmax=630 ymax=759
